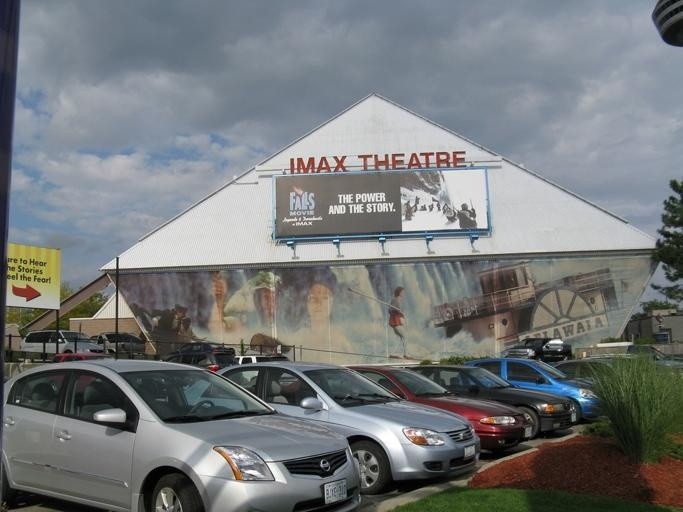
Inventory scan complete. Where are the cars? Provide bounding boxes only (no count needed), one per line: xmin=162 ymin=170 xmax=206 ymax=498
xmin=163 ymin=341 xmax=290 ymax=369
xmin=217 ymin=361 xmax=481 ymax=496
xmin=346 ymin=365 xmax=525 ymax=457
xmin=2 ymin=360 xmax=362 ymax=511
xmin=459 ymin=358 xmax=603 ymax=418
xmin=412 ymin=364 xmax=580 ymax=439
xmin=501 ymin=335 xmax=571 ymax=360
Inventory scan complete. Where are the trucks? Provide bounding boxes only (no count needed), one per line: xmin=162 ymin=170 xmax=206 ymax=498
xmin=575 ymin=343 xmax=683 ymax=370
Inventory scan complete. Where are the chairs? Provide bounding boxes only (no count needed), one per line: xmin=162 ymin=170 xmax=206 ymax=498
xmin=270 ymin=379 xmax=289 ymax=404
xmin=77 ymin=380 xmax=175 ymax=424
xmin=27 ymin=382 xmax=63 ymax=412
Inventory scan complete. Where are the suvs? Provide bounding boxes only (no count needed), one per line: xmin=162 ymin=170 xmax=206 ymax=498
xmin=20 ymin=329 xmax=144 ymax=362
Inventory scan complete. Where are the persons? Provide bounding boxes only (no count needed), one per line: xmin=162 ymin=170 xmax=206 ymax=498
xmin=281 ymin=277 xmax=350 ymax=368
xmin=206 ymin=271 xmax=281 ymax=355
xmin=388 ymin=286 xmax=410 ymax=359
xmin=457 ymin=203 xmax=477 ymax=229
xmin=150 ymin=304 xmax=206 ymax=354
